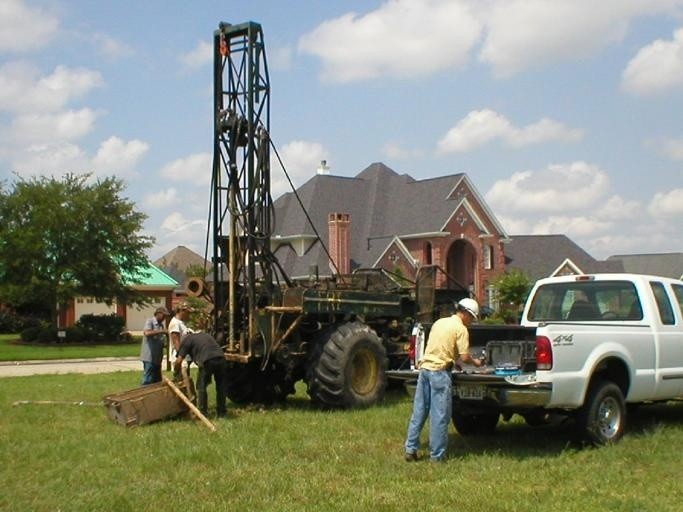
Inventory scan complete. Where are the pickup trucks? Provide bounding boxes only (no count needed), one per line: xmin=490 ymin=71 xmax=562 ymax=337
xmin=381 ymin=271 xmax=683 ymax=447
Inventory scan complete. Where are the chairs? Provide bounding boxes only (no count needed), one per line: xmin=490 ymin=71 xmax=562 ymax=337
xmin=566 ymin=301 xmax=602 ymax=320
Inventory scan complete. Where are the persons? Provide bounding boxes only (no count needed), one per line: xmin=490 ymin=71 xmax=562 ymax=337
xmin=168 ymin=301 xmax=195 ymax=385
xmin=402 ymin=297 xmax=486 ymax=462
xmin=173 ymin=328 xmax=228 ymax=419
xmin=139 ymin=307 xmax=171 ymax=385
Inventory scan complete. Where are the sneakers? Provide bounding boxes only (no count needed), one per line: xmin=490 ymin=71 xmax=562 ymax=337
xmin=405 ymin=454 xmax=449 ymax=463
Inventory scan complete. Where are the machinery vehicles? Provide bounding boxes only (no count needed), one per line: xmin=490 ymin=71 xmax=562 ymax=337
xmin=186 ymin=20 xmax=475 ymax=414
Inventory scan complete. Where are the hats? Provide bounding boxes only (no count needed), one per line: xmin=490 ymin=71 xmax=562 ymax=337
xmin=176 ymin=303 xmax=195 ymax=313
xmin=156 ymin=306 xmax=170 ymax=315
xmin=182 ymin=328 xmax=194 ymax=336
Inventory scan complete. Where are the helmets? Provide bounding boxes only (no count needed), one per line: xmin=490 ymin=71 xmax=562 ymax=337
xmin=459 ymin=298 xmax=480 ymax=321
